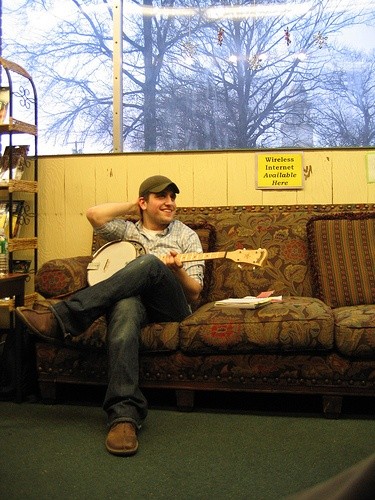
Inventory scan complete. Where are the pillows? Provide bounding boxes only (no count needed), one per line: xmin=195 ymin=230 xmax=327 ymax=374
xmin=306 ymin=212 xmax=375 ymax=309
xmin=185 ymin=223 xmax=217 ymax=310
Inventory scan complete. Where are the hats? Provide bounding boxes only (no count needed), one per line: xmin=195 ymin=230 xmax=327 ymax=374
xmin=139 ymin=175 xmax=179 ymax=197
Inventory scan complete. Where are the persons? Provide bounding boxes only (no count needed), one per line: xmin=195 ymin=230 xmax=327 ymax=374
xmin=15 ymin=173 xmax=206 ymax=457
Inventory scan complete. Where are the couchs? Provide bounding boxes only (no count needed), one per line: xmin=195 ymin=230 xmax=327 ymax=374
xmin=38 ymin=203 xmax=375 ymax=419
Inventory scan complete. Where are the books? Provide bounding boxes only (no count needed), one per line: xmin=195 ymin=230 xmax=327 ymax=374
xmin=214 ymin=290 xmax=281 ymax=309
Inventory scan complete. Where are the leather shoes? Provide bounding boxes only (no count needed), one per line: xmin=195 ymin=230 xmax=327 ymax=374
xmin=16 ymin=305 xmax=67 ymax=341
xmin=104 ymin=423 xmax=139 ymax=455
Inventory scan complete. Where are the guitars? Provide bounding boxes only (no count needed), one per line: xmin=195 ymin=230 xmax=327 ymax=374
xmin=86 ymin=240 xmax=268 ymax=287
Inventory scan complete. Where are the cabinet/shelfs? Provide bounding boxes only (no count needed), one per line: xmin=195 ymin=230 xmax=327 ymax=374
xmin=0 ymin=58 xmax=39 ymax=401
